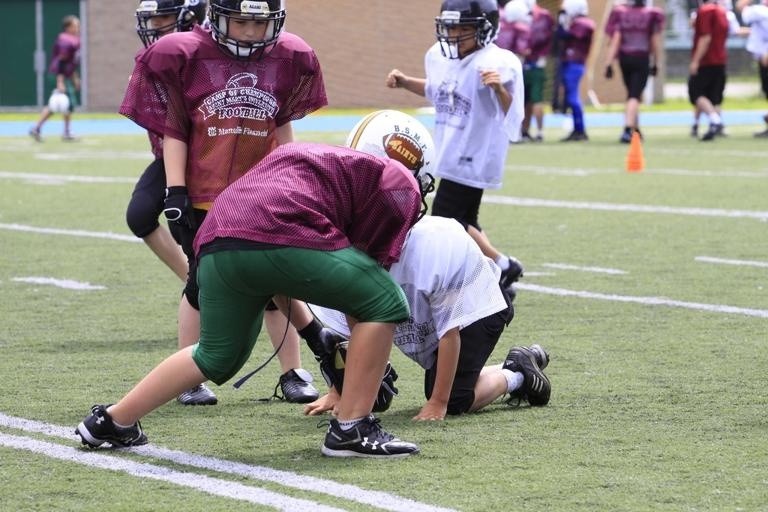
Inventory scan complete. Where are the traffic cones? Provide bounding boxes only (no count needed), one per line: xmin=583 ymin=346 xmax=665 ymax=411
xmin=626 ymin=131 xmax=645 ymax=174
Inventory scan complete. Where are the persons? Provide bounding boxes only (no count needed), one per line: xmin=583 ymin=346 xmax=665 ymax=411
xmin=385 ymin=0 xmax=524 ymax=302
xmin=75 ymin=109 xmax=437 ymax=457
xmin=135 ymin=0 xmax=208 ymax=281
xmin=119 ymin=0 xmax=327 ymax=402
xmin=303 ymin=215 xmax=552 ymax=409
xmin=30 ymin=13 xmax=81 ymax=142
xmin=495 ymin=0 xmax=768 ymax=144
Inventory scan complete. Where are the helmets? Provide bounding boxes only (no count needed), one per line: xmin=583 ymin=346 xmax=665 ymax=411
xmin=561 ymin=0 xmax=589 ymax=17
xmin=134 ymin=0 xmax=206 ymax=48
xmin=206 ymin=0 xmax=286 ymax=63
xmin=346 ymin=108 xmax=437 ymax=221
xmin=503 ymin=0 xmax=531 ymax=24
xmin=435 ymin=0 xmax=500 ymax=60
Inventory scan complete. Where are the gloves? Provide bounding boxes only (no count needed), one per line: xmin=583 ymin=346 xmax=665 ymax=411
xmin=164 ymin=185 xmax=199 ymax=247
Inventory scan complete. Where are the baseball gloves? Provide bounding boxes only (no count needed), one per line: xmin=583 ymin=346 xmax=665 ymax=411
xmin=318 ymin=335 xmax=401 ymax=413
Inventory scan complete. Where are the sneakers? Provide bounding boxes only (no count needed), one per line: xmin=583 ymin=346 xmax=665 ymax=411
xmin=499 ymin=255 xmax=524 ymax=289
xmin=561 ymin=130 xmax=588 ymax=141
xmin=75 ymin=404 xmax=149 ymax=449
xmin=279 ymin=368 xmax=319 ymax=404
xmin=701 ymin=123 xmax=724 ymax=141
xmin=317 ymin=413 xmax=421 ymax=458
xmin=177 ymin=383 xmax=218 ymax=405
xmin=502 ymin=344 xmax=552 ymax=406
xmin=509 ymin=134 xmax=531 ymax=144
